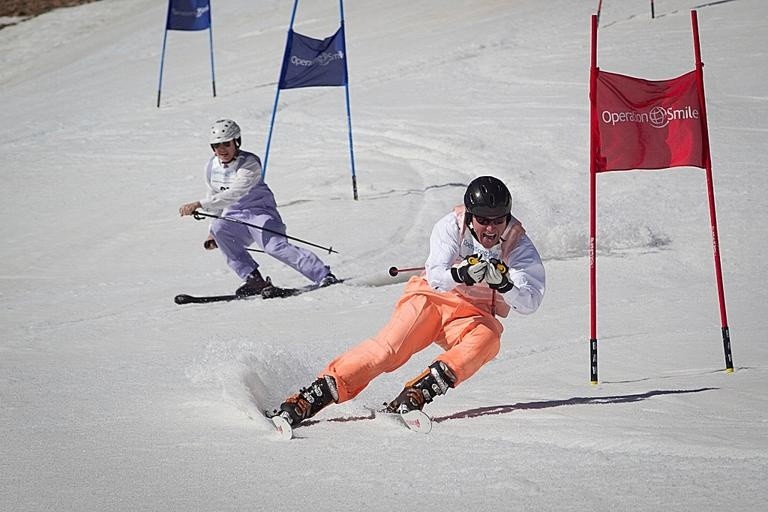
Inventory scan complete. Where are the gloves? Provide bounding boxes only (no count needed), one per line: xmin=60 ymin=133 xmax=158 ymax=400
xmin=485 ymin=257 xmax=515 ymax=293
xmin=450 ymin=251 xmax=488 ymax=287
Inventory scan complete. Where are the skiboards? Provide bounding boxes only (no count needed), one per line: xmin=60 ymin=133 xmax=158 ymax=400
xmin=269 ymin=405 xmax=434 ymax=441
xmin=174 ymin=277 xmax=359 ymax=305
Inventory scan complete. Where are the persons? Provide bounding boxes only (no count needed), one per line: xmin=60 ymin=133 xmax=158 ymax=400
xmin=178 ymin=119 xmax=337 ymax=295
xmin=281 ymin=175 xmax=547 ymax=423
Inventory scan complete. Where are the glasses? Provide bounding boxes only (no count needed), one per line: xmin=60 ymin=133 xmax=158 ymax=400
xmin=212 ymin=141 xmax=232 ymax=150
xmin=471 ymin=214 xmax=509 ymax=225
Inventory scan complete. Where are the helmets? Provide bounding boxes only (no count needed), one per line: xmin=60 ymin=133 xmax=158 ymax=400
xmin=208 ymin=117 xmax=241 ymax=145
xmin=464 ymin=175 xmax=513 ymax=220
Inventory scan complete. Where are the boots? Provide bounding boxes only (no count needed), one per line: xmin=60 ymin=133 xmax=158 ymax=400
xmin=318 ymin=272 xmax=338 ymax=287
xmin=386 ymin=360 xmax=459 ymax=415
xmin=280 ymin=374 xmax=339 ymax=427
xmin=235 ymin=268 xmax=268 ymax=296
